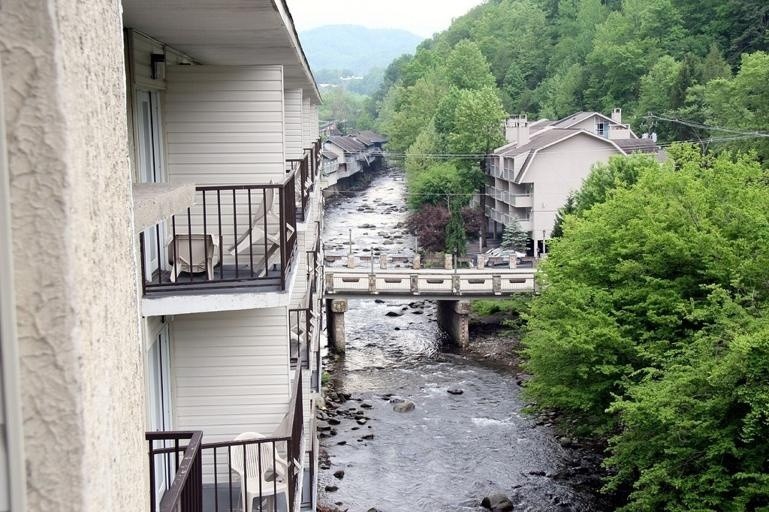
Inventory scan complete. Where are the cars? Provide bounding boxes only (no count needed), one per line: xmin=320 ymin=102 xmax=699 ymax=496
xmin=481 ymin=239 xmax=546 ymax=267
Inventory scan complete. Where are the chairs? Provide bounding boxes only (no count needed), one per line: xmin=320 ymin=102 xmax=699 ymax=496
xmin=291 ymin=322 xmax=309 ymax=358
xmin=230 ymin=431 xmax=289 ymax=512
xmin=227 ymin=180 xmax=295 ymax=279
xmin=167 ymin=234 xmax=220 ymax=283
